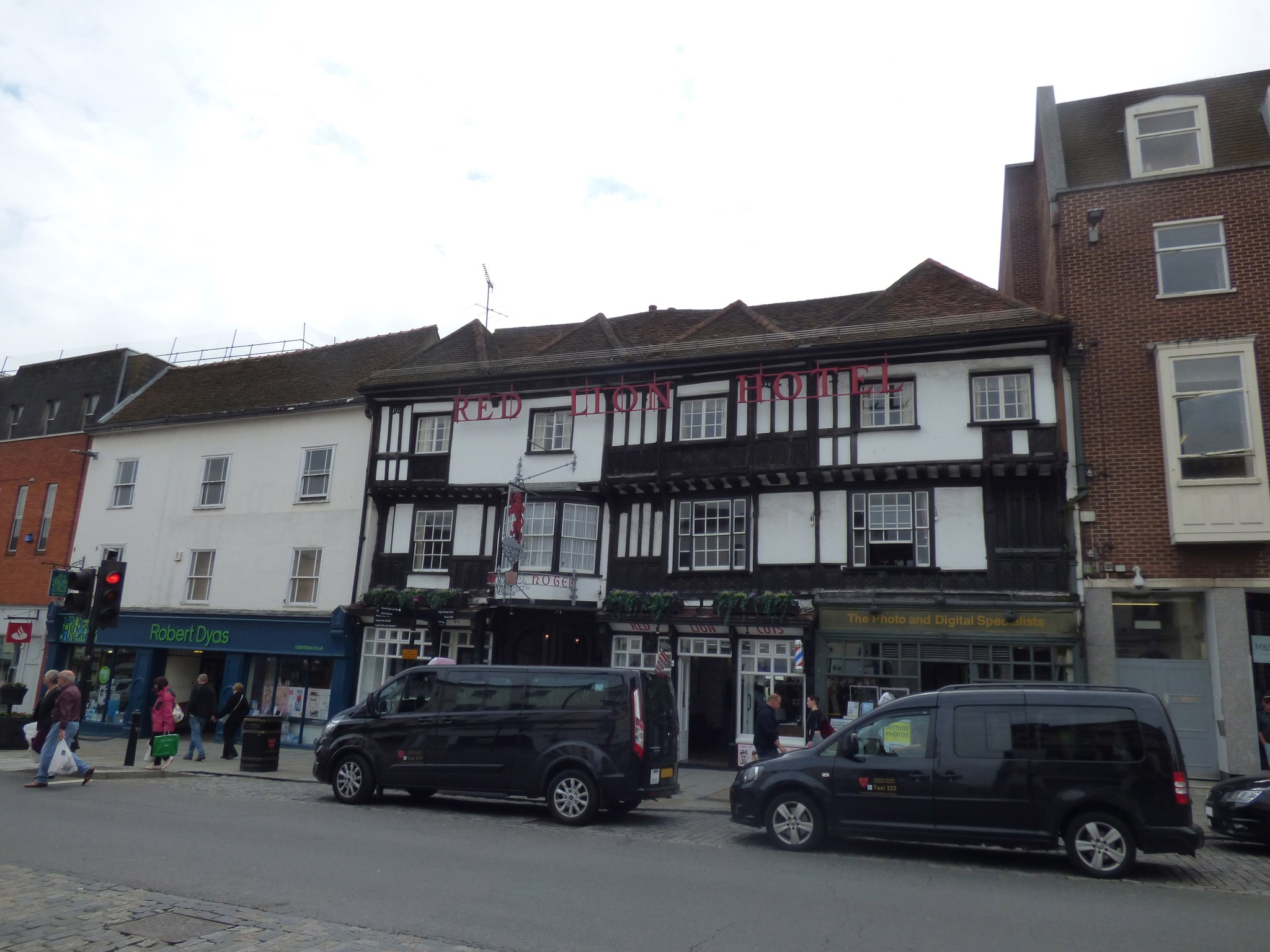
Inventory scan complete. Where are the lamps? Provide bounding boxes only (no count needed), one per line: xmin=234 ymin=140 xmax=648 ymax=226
xmin=656 ymin=624 xmax=660 ymax=634
xmin=870 ymin=590 xmax=881 ymax=615
xmin=1004 ymin=592 xmax=1019 ymax=624
xmin=1086 ymin=206 xmax=1105 ymax=244
xmin=932 ymin=583 xmax=946 ymax=605
xmin=71 ymin=449 xmax=100 ymax=460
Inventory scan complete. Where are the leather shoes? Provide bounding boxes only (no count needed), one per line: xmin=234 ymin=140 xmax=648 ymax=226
xmin=82 ymin=767 xmax=95 ymax=785
xmin=25 ymin=781 xmax=39 ymax=787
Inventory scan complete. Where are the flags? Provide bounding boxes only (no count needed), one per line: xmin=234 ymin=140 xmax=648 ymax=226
xmin=654 ymin=651 xmax=669 ymax=673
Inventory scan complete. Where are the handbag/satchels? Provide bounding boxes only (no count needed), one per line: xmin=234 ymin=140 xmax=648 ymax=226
xmin=219 ymin=714 xmax=229 ymax=723
xmin=30 ymin=750 xmax=41 ymax=763
xmin=71 ymin=732 xmax=81 ymax=750
xmin=820 ymin=716 xmax=836 ymax=739
xmin=23 ymin=722 xmax=38 ymax=752
xmin=152 ymin=730 xmax=180 ymax=757
xmin=48 ymin=738 xmax=78 ymax=776
xmin=172 ymin=703 xmax=184 ymax=723
xmin=143 ymin=740 xmax=156 ymax=762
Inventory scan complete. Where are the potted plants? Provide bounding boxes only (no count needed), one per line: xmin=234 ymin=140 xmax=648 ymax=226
xmin=713 ymin=591 xmax=800 ymax=624
xmin=602 ymin=588 xmax=683 ymax=620
xmin=361 ymin=585 xmax=467 ymax=608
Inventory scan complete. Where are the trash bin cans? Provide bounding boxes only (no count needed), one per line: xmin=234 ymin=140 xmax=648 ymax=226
xmin=240 ymin=713 xmax=282 ymax=773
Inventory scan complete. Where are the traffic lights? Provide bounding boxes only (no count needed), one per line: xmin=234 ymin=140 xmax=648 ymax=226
xmin=92 ymin=560 xmax=127 ymax=629
xmin=63 ymin=569 xmax=96 ymax=618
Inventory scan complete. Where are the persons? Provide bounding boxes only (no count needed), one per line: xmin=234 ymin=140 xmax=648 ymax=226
xmin=145 ymin=677 xmax=176 ymax=770
xmin=806 ymin=695 xmax=824 ymax=749
xmin=24 ymin=670 xmax=95 ymax=788
xmin=753 ymin=693 xmax=786 ymax=759
xmin=1257 ymin=695 xmax=1270 ymax=768
xmin=183 ymin=674 xmax=250 ymax=761
xmin=892 ymin=733 xmax=927 ymax=752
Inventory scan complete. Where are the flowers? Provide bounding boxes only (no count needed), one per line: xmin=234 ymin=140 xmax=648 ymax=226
xmin=0 ymin=709 xmax=35 ymax=717
xmin=0 ymin=681 xmax=28 ymax=689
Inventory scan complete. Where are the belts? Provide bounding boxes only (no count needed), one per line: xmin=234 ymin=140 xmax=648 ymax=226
xmin=70 ymin=720 xmax=79 ymax=722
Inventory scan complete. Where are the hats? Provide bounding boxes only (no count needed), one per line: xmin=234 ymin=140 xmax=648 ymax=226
xmin=1263 ymin=696 xmax=1270 ymax=702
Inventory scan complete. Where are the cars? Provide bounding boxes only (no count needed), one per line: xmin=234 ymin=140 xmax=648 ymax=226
xmin=1206 ymin=770 xmax=1270 ymax=843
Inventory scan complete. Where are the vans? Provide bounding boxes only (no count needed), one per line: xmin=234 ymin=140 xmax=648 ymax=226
xmin=312 ymin=664 xmax=681 ymax=827
xmin=730 ymin=680 xmax=1207 ymax=880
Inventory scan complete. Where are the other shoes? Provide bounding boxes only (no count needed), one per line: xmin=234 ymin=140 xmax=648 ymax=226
xmin=195 ymin=755 xmax=205 ymax=761
xmin=227 ymin=753 xmax=238 ymax=760
xmin=35 ymin=775 xmax=55 ymax=779
xmin=162 ymin=756 xmax=174 ymax=770
xmin=221 ymin=756 xmax=227 ymax=758
xmin=183 ymin=754 xmax=192 ymax=760
xmin=146 ymin=764 xmax=161 ymax=770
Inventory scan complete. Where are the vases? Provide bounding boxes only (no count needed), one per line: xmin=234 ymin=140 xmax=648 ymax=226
xmin=0 ymin=717 xmax=37 ymax=751
xmin=0 ymin=688 xmax=29 ymax=705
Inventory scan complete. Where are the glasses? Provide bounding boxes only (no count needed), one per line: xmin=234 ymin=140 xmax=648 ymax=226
xmin=57 ymin=677 xmax=64 ymax=679
xmin=1263 ymin=701 xmax=1270 ymax=704
xmin=44 ymin=676 xmax=46 ymax=678
xmin=154 ymin=684 xmax=159 ymax=688
